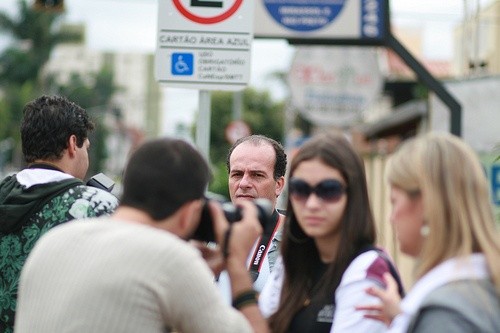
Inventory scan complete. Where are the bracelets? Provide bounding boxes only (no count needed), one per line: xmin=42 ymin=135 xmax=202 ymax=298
xmin=231 ymin=289 xmax=261 ymax=309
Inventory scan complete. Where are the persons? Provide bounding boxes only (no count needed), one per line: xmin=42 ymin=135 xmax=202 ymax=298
xmin=13 ymin=137 xmax=270 ymax=333
xmin=206 ymin=134 xmax=285 ymax=320
xmin=355 ymin=129 xmax=500 ymax=333
xmin=0 ymin=95 xmax=120 ymax=333
xmin=266 ymin=132 xmax=406 ymax=333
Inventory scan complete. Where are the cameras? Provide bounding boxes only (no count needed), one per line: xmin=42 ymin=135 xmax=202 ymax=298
xmin=192 ymin=192 xmax=272 ymax=243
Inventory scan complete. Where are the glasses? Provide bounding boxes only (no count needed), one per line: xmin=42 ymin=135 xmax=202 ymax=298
xmin=287 ymin=178 xmax=351 ymax=199
